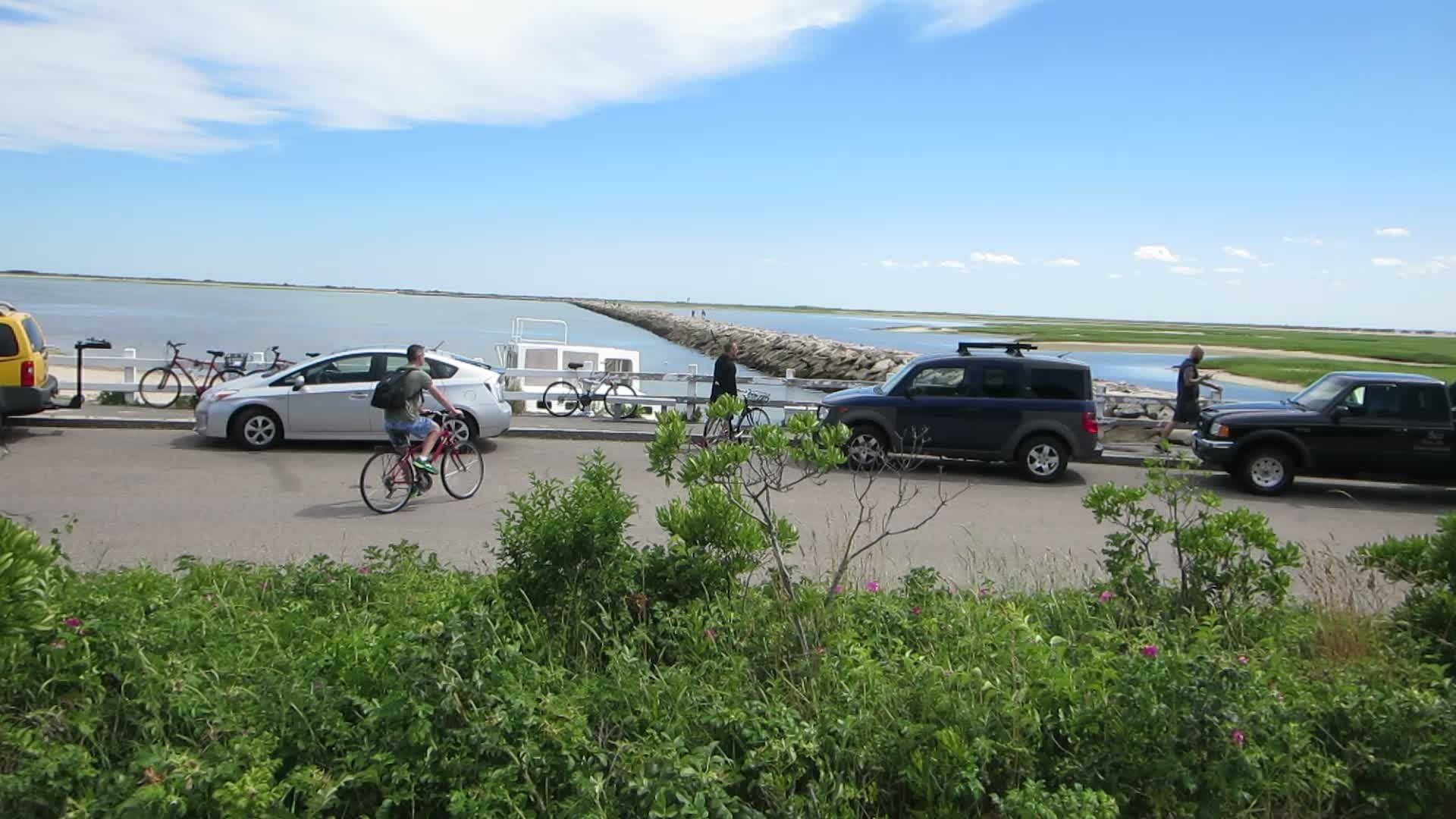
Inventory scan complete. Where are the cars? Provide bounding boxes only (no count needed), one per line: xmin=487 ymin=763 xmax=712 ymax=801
xmin=193 ymin=344 xmax=514 ymax=451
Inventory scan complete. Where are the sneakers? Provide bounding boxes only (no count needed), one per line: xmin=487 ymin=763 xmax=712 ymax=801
xmin=413 ymin=456 xmax=438 ymax=475
xmin=406 ymin=488 xmax=419 ymax=499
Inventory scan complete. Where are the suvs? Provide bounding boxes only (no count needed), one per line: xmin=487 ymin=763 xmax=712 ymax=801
xmin=811 ymin=340 xmax=1099 ymax=483
xmin=0 ymin=300 xmax=61 ymax=417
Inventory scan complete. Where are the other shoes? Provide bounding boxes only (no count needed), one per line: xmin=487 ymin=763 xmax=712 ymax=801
xmin=1154 ymin=444 xmax=1171 ymax=453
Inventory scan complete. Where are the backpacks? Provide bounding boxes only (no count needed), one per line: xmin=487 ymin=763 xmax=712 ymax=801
xmin=371 ymin=368 xmax=425 ymax=410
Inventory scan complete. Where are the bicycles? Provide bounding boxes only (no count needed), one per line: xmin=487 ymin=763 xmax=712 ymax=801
xmin=359 ymin=410 xmax=485 ymax=515
xmin=541 ymin=359 xmax=640 ymax=420
xmin=702 ymin=389 xmax=771 ymax=452
xmin=243 ymin=344 xmax=320 ymax=375
xmin=137 ymin=340 xmax=246 ymax=410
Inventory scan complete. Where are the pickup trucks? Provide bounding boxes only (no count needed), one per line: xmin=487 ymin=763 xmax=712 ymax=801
xmin=1187 ymin=370 xmax=1456 ymax=498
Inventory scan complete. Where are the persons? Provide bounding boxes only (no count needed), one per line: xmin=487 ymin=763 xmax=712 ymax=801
xmin=1155 ymin=344 xmax=1214 ymax=455
xmin=710 ymin=342 xmax=739 ymax=441
xmin=384 ymin=345 xmax=465 ymax=499
